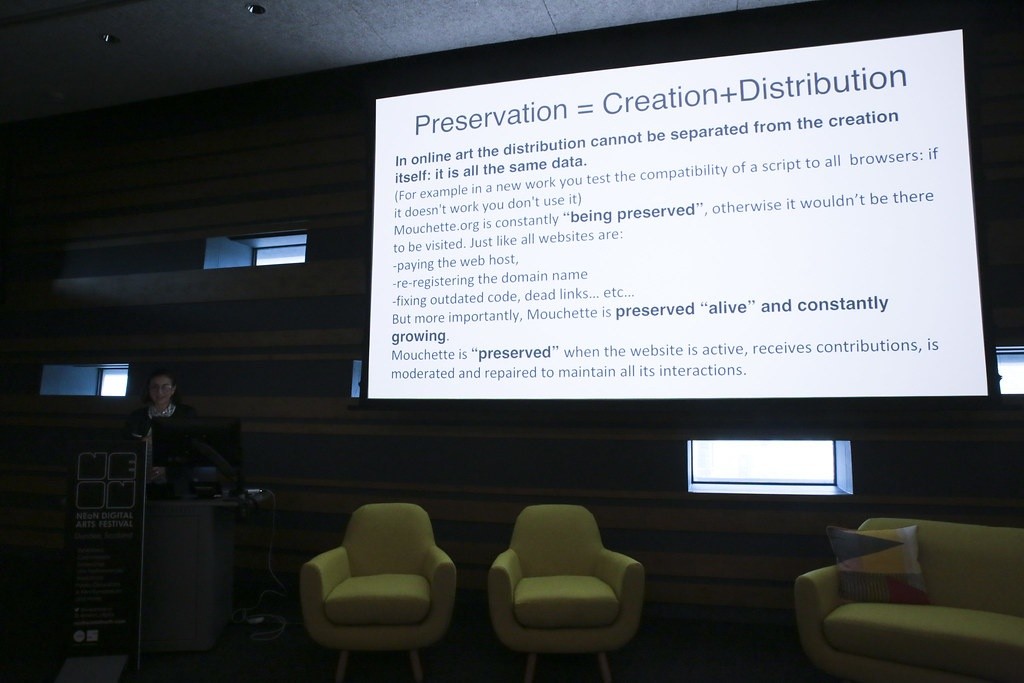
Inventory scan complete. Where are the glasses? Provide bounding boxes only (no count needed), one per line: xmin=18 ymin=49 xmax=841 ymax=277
xmin=148 ymin=383 xmax=174 ymax=397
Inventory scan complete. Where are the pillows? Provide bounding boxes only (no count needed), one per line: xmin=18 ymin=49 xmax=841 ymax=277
xmin=824 ymin=524 xmax=935 ymax=604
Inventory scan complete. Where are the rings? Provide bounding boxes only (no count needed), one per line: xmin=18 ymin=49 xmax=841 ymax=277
xmin=155 ymin=471 xmax=159 ymax=473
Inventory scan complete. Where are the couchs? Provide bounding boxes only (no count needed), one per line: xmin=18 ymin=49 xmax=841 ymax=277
xmin=794 ymin=516 xmax=1024 ymax=683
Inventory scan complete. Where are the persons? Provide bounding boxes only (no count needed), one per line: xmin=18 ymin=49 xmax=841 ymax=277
xmin=120 ymin=367 xmax=206 ymax=498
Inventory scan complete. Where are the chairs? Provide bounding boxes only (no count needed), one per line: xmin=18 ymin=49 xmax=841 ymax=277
xmin=299 ymin=503 xmax=456 ymax=683
xmin=488 ymin=504 xmax=644 ymax=683
